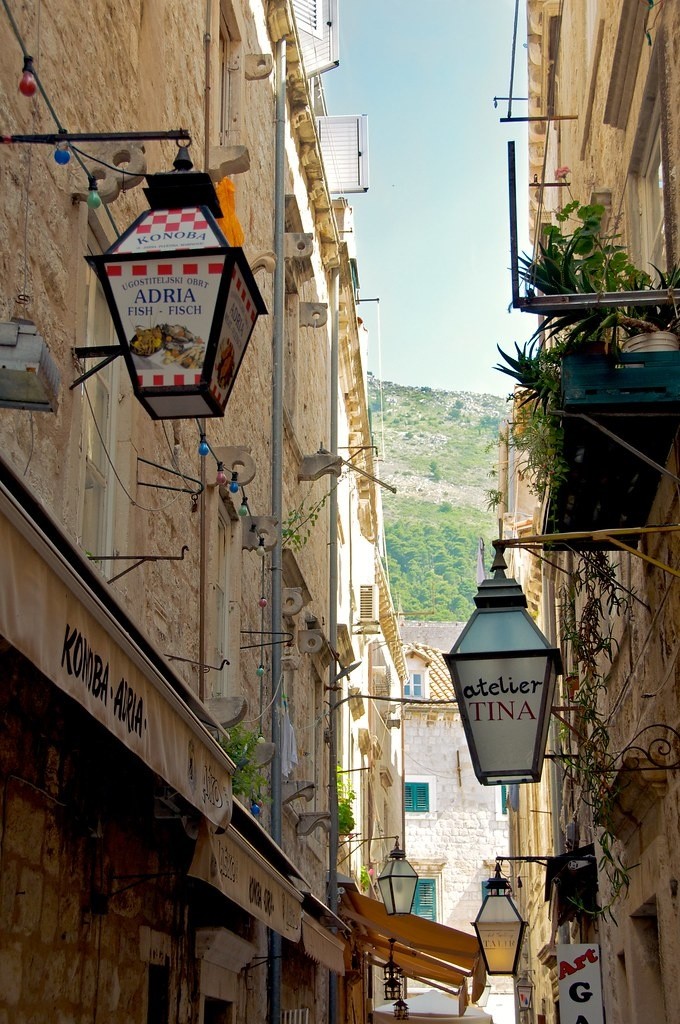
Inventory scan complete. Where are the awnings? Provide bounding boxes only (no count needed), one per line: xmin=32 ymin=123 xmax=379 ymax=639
xmin=186 ymin=808 xmax=304 ymax=944
xmin=302 ymin=902 xmax=346 ymax=979
xmin=0 ymin=446 xmax=236 ymax=836
xmin=343 ymin=876 xmax=487 ymax=1017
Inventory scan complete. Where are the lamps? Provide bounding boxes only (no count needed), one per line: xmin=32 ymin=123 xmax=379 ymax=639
xmin=337 ymin=836 xmax=419 ymax=916
xmin=516 ymin=970 xmax=535 ymax=1011
xmin=0 ymin=130 xmax=269 ymax=420
xmin=441 ymin=526 xmax=680 ymax=786
xmin=469 ymin=856 xmax=551 ymax=977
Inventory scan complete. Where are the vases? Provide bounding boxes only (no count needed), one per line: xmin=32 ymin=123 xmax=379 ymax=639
xmin=622 ymin=332 xmax=679 ymax=369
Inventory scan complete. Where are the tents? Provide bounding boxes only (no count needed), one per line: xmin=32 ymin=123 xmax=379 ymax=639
xmin=372 ymin=989 xmax=494 ymax=1024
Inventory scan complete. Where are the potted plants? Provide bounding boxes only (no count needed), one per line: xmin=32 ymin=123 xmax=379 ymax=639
xmin=360 ymin=865 xmax=370 ymax=893
xmin=336 ymin=766 xmax=357 ymax=841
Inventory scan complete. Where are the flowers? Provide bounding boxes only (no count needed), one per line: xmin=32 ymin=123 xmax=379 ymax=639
xmin=482 ymin=165 xmax=680 ymax=935
